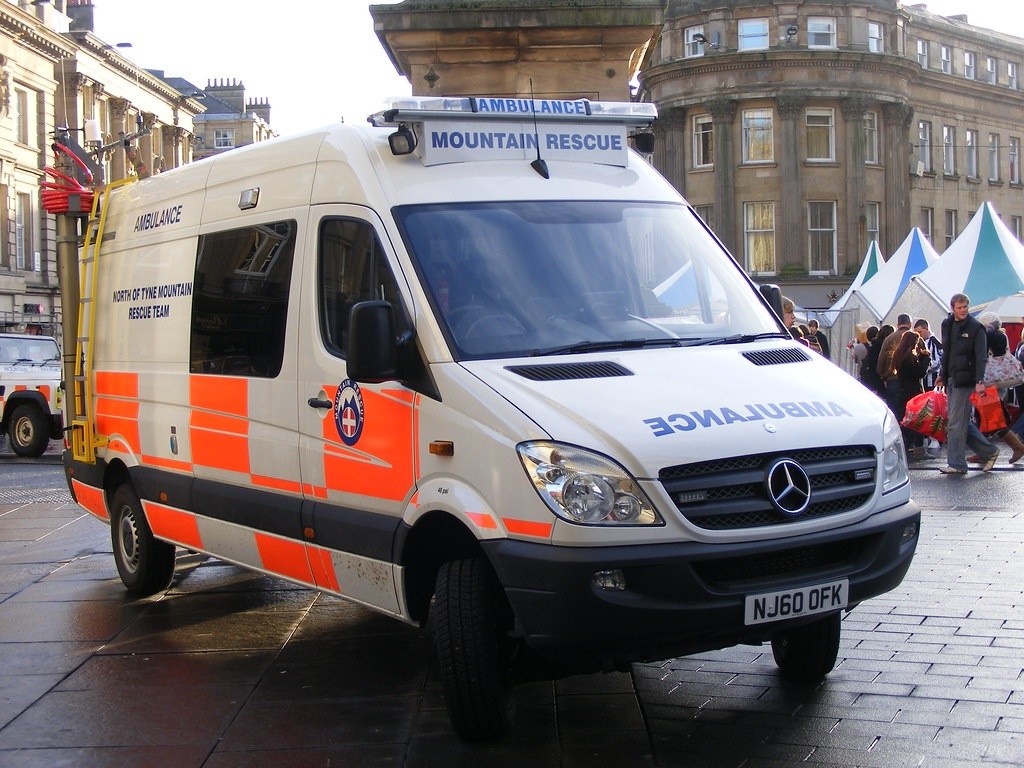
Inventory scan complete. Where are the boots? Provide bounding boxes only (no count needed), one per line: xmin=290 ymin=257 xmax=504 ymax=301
xmin=966 ymin=454 xmax=984 ymax=463
xmin=1001 ymin=430 xmax=1024 ymax=464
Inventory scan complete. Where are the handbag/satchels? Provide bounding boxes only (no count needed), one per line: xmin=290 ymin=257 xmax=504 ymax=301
xmin=968 ymin=386 xmax=1012 ymax=438
xmin=983 ymin=353 xmax=1024 ymax=386
xmin=900 ymin=385 xmax=949 ymax=443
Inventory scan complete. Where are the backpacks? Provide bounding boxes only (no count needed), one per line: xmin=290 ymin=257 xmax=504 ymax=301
xmin=860 ymin=341 xmax=878 ymax=391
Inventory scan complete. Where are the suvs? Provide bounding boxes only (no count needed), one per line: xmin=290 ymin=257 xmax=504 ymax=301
xmin=0 ymin=335 xmax=62 ymax=458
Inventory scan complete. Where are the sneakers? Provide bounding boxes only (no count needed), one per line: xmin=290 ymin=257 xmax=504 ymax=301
xmin=913 ymin=446 xmax=936 ymax=459
xmin=982 ymin=446 xmax=1000 ymax=472
xmin=922 ymin=438 xmax=929 ymax=448
xmin=939 ymin=466 xmax=969 ymax=473
xmin=927 ymin=436 xmax=941 ymax=449
xmin=908 ymin=445 xmax=916 ymax=451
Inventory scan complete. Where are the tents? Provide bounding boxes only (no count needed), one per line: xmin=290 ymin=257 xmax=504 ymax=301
xmin=807 ymin=198 xmax=1024 ymax=381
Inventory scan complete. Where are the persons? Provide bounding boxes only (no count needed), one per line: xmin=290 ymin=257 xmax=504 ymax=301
xmin=790 ymin=326 xmax=808 ymax=345
xmin=808 ymin=319 xmax=830 ymax=360
xmin=799 ymin=325 xmax=823 ymax=357
xmin=891 ymin=331 xmax=934 ymax=459
xmin=934 ymin=294 xmax=1000 ymax=474
xmin=781 ymin=295 xmax=796 ymax=329
xmin=852 ymin=325 xmax=878 ymax=382
xmin=874 ymin=313 xmax=927 ymax=452
xmin=860 ymin=325 xmax=893 ymax=395
xmin=966 ymin=310 xmax=1024 ymax=464
xmin=913 ymin=318 xmax=943 ymax=449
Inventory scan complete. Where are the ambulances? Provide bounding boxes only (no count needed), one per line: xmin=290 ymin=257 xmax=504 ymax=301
xmin=63 ymin=95 xmax=920 ymax=746
